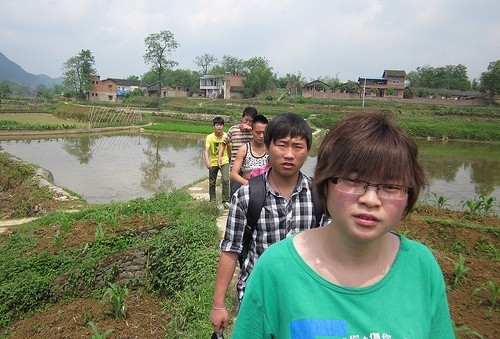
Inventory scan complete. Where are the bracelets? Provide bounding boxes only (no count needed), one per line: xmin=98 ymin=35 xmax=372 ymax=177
xmin=213 ymin=308 xmax=225 ymax=310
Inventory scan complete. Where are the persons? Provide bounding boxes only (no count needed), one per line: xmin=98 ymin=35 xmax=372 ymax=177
xmin=217 ymin=106 xmax=257 ymax=197
xmin=229 ymin=112 xmax=456 ymax=339
xmin=211 ymin=113 xmax=332 ymax=333
xmin=231 ymin=115 xmax=269 ymax=185
xmin=205 ymin=117 xmax=232 ymax=208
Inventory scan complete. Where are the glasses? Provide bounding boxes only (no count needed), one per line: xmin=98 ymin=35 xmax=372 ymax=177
xmin=328 ymin=176 xmax=415 ymax=200
